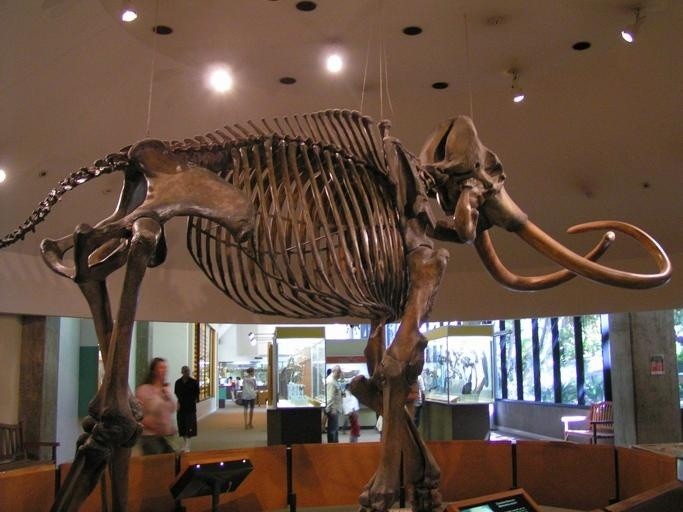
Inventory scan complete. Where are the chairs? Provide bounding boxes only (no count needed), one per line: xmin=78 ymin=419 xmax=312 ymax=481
xmin=560 ymin=400 xmax=614 ymax=444
xmin=0 ymin=420 xmax=60 ymax=470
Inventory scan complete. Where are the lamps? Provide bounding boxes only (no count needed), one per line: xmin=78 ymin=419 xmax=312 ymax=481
xmin=620 ymin=6 xmax=646 ymax=44
xmin=507 ymin=67 xmax=525 ymax=104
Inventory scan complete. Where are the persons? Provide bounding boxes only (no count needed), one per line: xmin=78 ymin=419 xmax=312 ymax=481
xmin=173 ymin=364 xmax=200 ymax=454
xmin=414 ymin=370 xmax=426 ymax=431
xmin=227 ymin=376 xmax=235 ymax=402
xmin=234 ymin=376 xmax=240 ymax=392
xmin=136 ymin=356 xmax=180 ymax=457
xmin=242 ymin=366 xmax=256 ymax=430
xmin=320 ymin=365 xmax=361 ymax=442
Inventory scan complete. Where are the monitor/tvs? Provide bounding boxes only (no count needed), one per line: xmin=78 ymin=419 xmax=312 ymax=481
xmin=447 ymin=489 xmax=541 ymax=512
